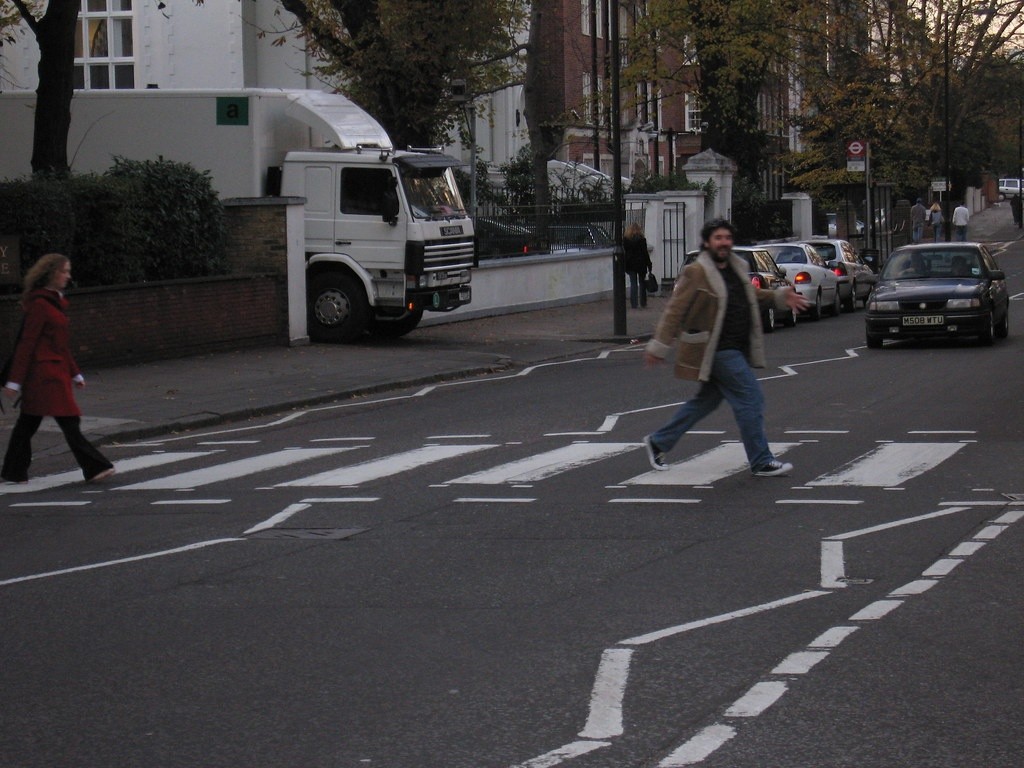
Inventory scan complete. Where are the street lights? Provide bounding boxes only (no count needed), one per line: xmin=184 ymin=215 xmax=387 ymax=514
xmin=944 ymin=10 xmax=998 ymax=263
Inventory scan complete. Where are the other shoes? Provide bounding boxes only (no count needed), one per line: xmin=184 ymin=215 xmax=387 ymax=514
xmin=0 ymin=470 xmax=30 ymax=485
xmin=83 ymin=462 xmax=116 ymax=483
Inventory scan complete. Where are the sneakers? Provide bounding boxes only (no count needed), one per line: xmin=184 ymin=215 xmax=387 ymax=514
xmin=643 ymin=434 xmax=670 ymax=471
xmin=751 ymin=459 xmax=794 ymax=477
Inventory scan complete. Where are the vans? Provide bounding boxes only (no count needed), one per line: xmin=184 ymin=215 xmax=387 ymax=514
xmin=998 ymin=178 xmax=1024 ymax=203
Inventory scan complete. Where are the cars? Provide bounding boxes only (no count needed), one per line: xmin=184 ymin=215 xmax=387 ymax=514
xmin=754 ymin=243 xmax=842 ymax=323
xmin=794 ymin=238 xmax=877 ymax=313
xmin=475 ymin=216 xmax=544 ymax=261
xmin=861 ymin=241 xmax=1011 ymax=349
xmin=925 ymin=209 xmax=945 ymax=223
xmin=522 ymin=224 xmax=617 ymax=254
xmin=676 ymin=247 xmax=797 ymax=334
xmin=826 ymin=214 xmax=864 ymax=237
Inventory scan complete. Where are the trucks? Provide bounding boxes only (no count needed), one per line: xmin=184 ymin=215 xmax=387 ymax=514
xmin=0 ymin=89 xmax=478 ymax=345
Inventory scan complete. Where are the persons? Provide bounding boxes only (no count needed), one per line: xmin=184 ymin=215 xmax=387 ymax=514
xmin=1010 ymin=194 xmax=1022 ymax=225
xmin=623 ymin=222 xmax=653 ymax=309
xmin=643 ymin=216 xmax=810 ymax=478
xmin=927 ymin=203 xmax=944 ymax=243
xmin=952 ymin=200 xmax=969 ymax=242
xmin=0 ymin=253 xmax=116 ymax=486
xmin=910 ymin=198 xmax=926 ymax=245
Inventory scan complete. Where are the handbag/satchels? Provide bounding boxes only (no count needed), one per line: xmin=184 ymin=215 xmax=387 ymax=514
xmin=646 ymin=273 xmax=659 ymax=293
